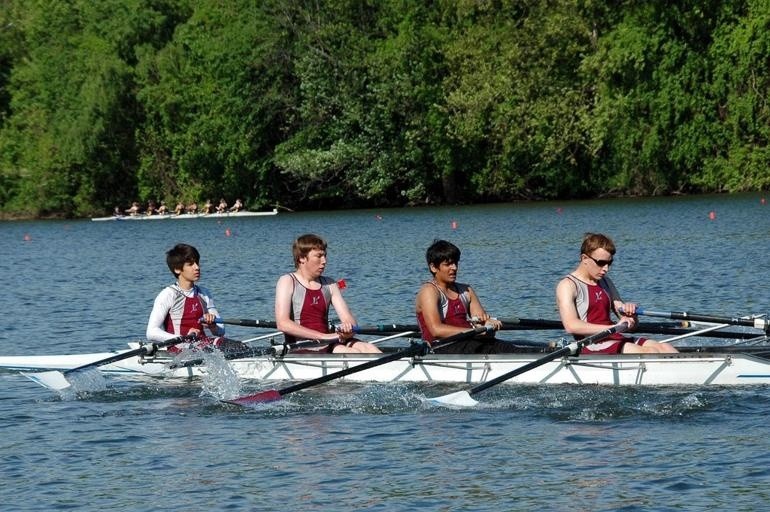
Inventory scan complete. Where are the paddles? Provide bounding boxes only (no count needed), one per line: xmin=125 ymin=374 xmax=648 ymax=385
xmin=19 ymin=333 xmax=195 ymax=391
xmin=424 ymin=322 xmax=625 ymax=408
xmin=472 ymin=319 xmax=727 ymax=328
xmin=220 ymin=323 xmax=491 ymax=410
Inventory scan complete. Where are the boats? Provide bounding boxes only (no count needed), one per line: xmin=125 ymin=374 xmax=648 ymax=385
xmin=0 ymin=321 xmax=770 ymax=398
xmin=90 ymin=206 xmax=279 ymax=221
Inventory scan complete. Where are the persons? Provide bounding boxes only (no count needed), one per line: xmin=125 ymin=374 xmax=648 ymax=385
xmin=274 ymin=233 xmax=383 ymax=354
xmin=555 ymin=231 xmax=682 ymax=355
xmin=144 ymin=243 xmax=249 ymax=356
xmin=415 ymin=238 xmax=525 ymax=352
xmin=112 ymin=198 xmax=243 ymax=217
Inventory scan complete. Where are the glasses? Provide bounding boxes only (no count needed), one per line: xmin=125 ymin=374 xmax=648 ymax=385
xmin=581 ymin=252 xmax=613 ymax=266
